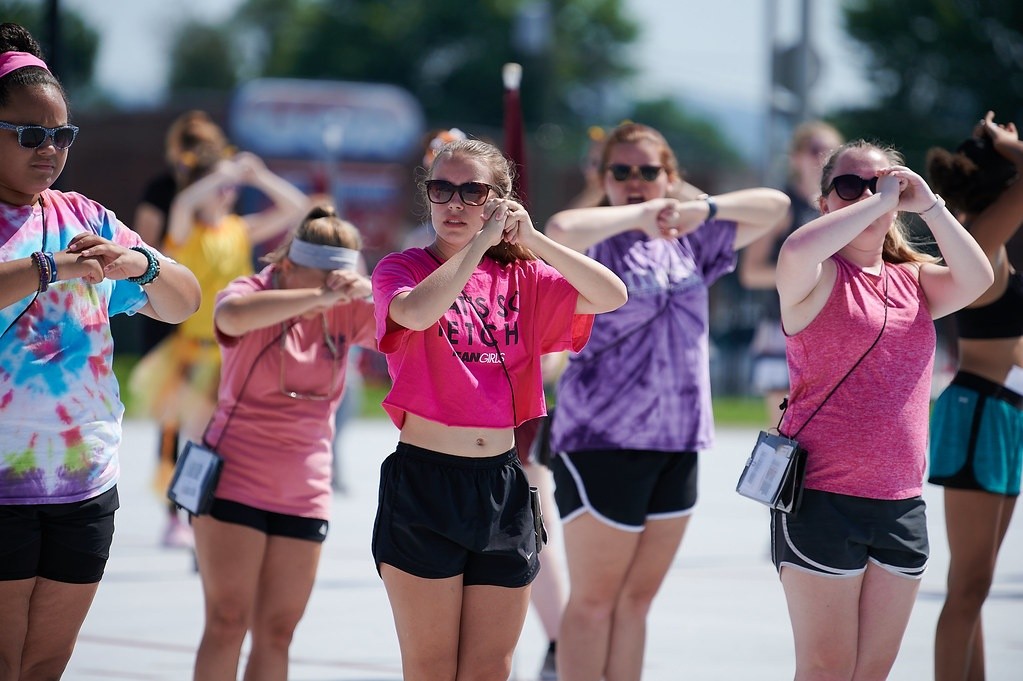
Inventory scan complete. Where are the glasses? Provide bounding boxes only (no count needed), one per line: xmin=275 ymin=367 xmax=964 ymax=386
xmin=824 ymin=173 xmax=878 ymax=201
xmin=608 ymin=163 xmax=665 ymax=183
xmin=0 ymin=121 xmax=79 ymax=150
xmin=424 ymin=180 xmax=504 ymax=206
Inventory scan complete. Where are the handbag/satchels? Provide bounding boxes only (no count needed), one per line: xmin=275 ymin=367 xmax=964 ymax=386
xmin=167 ymin=439 xmax=223 ymax=516
xmin=530 ymin=487 xmax=547 ymax=554
xmin=736 ymin=430 xmax=808 ymax=517
xmin=534 ymin=404 xmax=554 ymax=467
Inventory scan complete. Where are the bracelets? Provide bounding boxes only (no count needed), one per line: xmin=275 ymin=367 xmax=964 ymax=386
xmin=32 ymin=252 xmax=49 ymax=292
xmin=125 ymin=246 xmax=160 ymax=286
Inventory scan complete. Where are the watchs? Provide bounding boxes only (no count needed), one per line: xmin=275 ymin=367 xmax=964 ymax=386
xmin=44 ymin=252 xmax=57 ymax=283
xmin=918 ymin=194 xmax=946 ymax=222
xmin=695 ymin=194 xmax=718 ymax=227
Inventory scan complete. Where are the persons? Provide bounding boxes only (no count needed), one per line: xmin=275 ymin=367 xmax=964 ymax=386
xmin=403 ymin=128 xmax=566 ymax=681
xmin=372 ymin=139 xmax=629 ymax=680
xmin=134 ymin=110 xmax=309 ymax=552
xmin=741 ymin=122 xmax=846 ymax=434
xmin=1 ymin=22 xmax=201 ymax=681
xmin=545 ymin=120 xmax=792 ymax=681
xmin=770 ymin=139 xmax=995 ymax=681
xmin=188 ymin=204 xmax=377 ymax=681
xmin=304 ymin=194 xmax=359 ymax=500
xmin=927 ymin=111 xmax=1023 ymax=680
